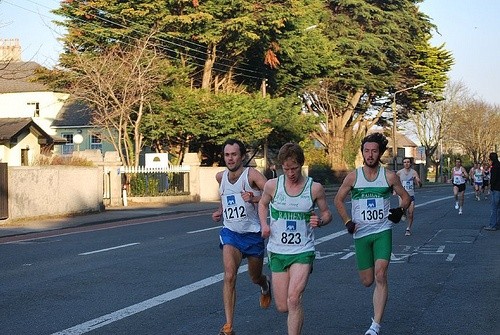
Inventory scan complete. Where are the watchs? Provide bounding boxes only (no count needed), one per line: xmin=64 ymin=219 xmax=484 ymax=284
xmin=320 ymin=219 xmax=323 ymax=224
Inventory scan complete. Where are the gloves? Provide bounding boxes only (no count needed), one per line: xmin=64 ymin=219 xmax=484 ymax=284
xmin=345 ymin=220 xmax=356 ymax=234
xmin=388 ymin=207 xmax=406 ymax=224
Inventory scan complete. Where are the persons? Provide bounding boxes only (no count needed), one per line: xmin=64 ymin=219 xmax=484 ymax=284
xmin=265 ymin=163 xmax=277 ymax=179
xmin=258 ymin=143 xmax=332 ymax=335
xmin=469 ymin=162 xmax=492 ymax=201
xmin=334 ymin=132 xmax=412 ymax=334
xmin=451 ymin=158 xmax=469 ymax=215
xmin=212 ymin=138 xmax=271 ymax=335
xmin=392 ymin=157 xmax=422 ymax=236
xmin=485 ymin=152 xmax=500 ymax=231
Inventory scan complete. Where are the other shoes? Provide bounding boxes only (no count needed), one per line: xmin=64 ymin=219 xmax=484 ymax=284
xmin=458 ymin=209 xmax=463 ymax=215
xmin=217 ymin=323 xmax=233 ymax=335
xmin=485 ymin=226 xmax=496 ymax=231
xmin=404 ymin=228 xmax=412 ymax=236
xmin=455 ymin=201 xmax=460 ymax=210
xmin=364 ymin=316 xmax=381 ymax=335
xmin=259 ymin=275 xmax=272 ymax=309
xmin=400 ymin=208 xmax=407 ymax=222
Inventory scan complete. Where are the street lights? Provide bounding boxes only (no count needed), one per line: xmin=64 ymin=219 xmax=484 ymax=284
xmin=72 ymin=134 xmax=84 ymax=152
xmin=392 ymin=82 xmax=428 ymax=172
